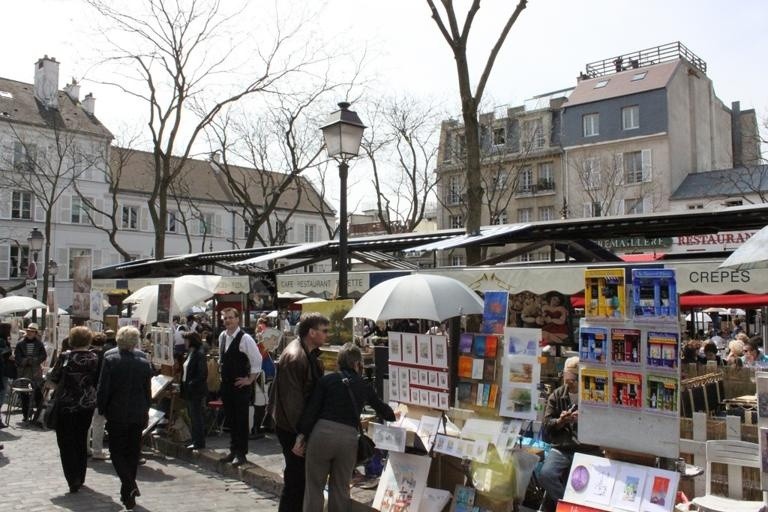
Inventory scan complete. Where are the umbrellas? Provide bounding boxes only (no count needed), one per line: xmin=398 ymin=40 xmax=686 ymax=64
xmin=340 ymin=275 xmax=490 ymax=323
xmin=720 ymin=226 xmax=768 ymax=268
xmin=0 ymin=294 xmax=49 ymax=319
xmin=117 ymin=275 xmax=219 ymax=323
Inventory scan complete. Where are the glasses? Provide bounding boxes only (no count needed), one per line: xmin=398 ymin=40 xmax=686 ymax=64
xmin=318 ymin=328 xmax=328 ymax=333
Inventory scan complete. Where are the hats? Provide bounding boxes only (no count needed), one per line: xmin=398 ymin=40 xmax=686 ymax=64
xmin=181 ymin=331 xmax=202 ymax=346
xmin=564 ymin=357 xmax=578 ymax=380
xmin=26 ymin=323 xmax=38 ymax=332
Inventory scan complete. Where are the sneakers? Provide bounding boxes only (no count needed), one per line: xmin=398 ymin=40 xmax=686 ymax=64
xmin=70 ymin=440 xmax=246 ymax=509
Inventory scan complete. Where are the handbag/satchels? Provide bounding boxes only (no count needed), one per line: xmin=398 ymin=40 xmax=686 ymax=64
xmin=33 ymin=386 xmax=64 ymax=430
xmin=358 ymin=433 xmax=375 ymax=461
xmin=206 ymin=357 xmax=220 ymax=392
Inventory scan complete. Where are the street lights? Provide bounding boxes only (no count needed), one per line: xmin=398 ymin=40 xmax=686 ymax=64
xmin=26 ymin=226 xmax=43 ymax=321
xmin=320 ymin=103 xmax=365 ymax=298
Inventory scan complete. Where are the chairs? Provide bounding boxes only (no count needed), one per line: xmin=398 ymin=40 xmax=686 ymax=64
xmin=5 ymin=378 xmax=34 ymax=425
xmin=693 ymin=440 xmax=768 ymax=512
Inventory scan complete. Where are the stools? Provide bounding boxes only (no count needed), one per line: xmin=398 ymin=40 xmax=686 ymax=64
xmin=208 ymin=400 xmax=226 ymax=436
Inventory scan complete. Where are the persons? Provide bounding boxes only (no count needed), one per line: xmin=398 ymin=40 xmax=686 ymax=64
xmin=687 ymin=317 xmax=768 ymax=373
xmin=47 ymin=327 xmax=154 ymax=508
xmin=162 ymin=304 xmax=281 ymax=467
xmin=273 ymin=314 xmax=329 ymax=512
xmin=540 ymin=355 xmax=582 ymax=503
xmin=507 ymin=290 xmax=569 ymax=347
xmin=0 ymin=320 xmax=12 ymax=430
xmin=15 ymin=324 xmax=47 ymax=423
xmin=294 ymin=341 xmax=395 ymax=511
